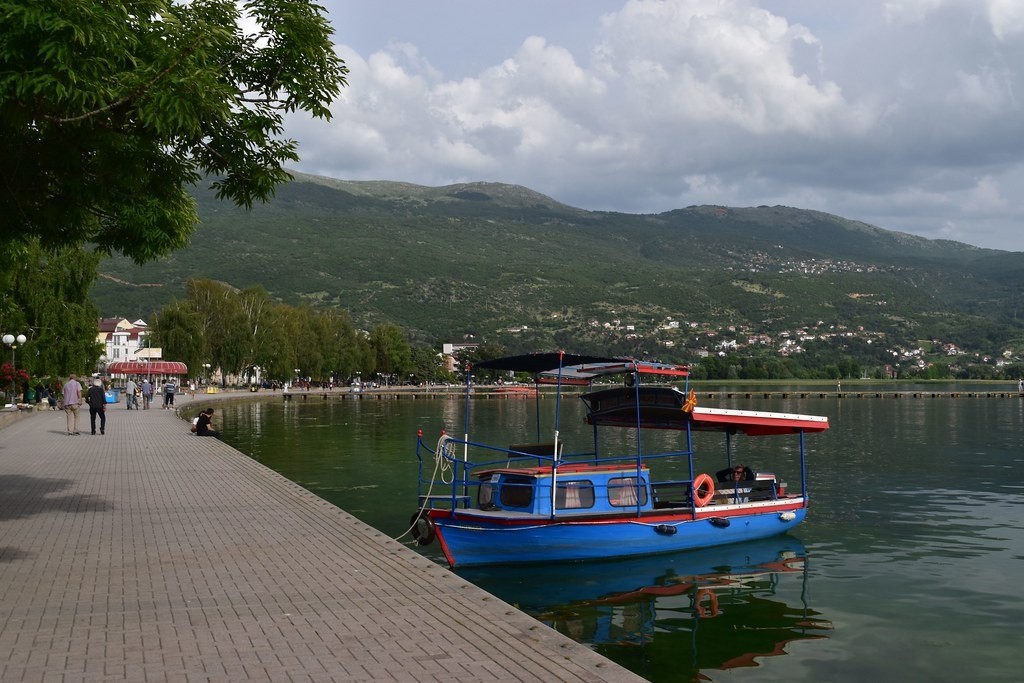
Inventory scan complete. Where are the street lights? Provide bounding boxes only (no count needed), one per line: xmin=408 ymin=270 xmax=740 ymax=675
xmin=294 ymin=368 xmax=301 ymax=388
xmin=202 ymin=363 xmax=211 ymax=388
xmin=2 ymin=334 xmax=26 ymax=405
xmin=138 ymin=359 xmax=147 ymax=382
xmin=99 ymin=354 xmax=113 ymax=391
xmin=253 ymin=366 xmax=261 ymax=390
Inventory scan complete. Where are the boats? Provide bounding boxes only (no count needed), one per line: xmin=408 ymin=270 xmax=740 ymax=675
xmin=450 ymin=530 xmax=834 ymax=683
xmin=410 ymin=350 xmax=833 ymax=571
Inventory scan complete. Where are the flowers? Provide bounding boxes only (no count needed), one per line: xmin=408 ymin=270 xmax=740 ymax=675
xmin=0 ymin=363 xmax=30 ymax=386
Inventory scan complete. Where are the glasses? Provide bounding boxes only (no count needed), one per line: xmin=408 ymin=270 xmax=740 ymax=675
xmin=734 ymin=470 xmax=742 ymax=474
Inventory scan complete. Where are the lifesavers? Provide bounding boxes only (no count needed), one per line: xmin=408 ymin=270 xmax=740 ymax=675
xmin=695 ymin=589 xmax=719 ymax=618
xmin=692 ymin=472 xmax=715 ymax=508
xmin=409 ymin=511 xmax=437 ymax=546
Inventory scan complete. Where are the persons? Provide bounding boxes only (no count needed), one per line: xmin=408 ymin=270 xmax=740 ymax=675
xmin=161 ymin=379 xmax=175 ymax=409
xmin=126 ymin=377 xmax=138 ymax=409
xmin=141 ymin=379 xmax=155 ymax=411
xmin=42 ymin=383 xmax=64 ymax=411
xmin=715 ymin=464 xmax=755 ymax=503
xmin=85 ymin=379 xmax=107 ymax=435
xmin=196 ymin=408 xmax=220 ymax=439
xmin=191 ymin=410 xmax=206 ymax=433
xmin=62 ymin=373 xmax=83 ymax=435
